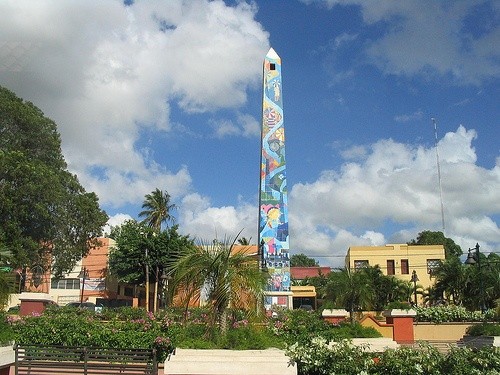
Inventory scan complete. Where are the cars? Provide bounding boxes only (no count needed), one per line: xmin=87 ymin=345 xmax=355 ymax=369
xmin=64 ymin=301 xmax=96 ymax=314
xmin=299 ymin=304 xmax=313 ymax=313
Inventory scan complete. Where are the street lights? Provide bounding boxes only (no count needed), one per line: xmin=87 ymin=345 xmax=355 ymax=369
xmin=409 ymin=269 xmax=420 ymax=308
xmin=464 ymin=243 xmax=488 ymax=323
xmin=77 ymin=265 xmax=90 ymax=310
